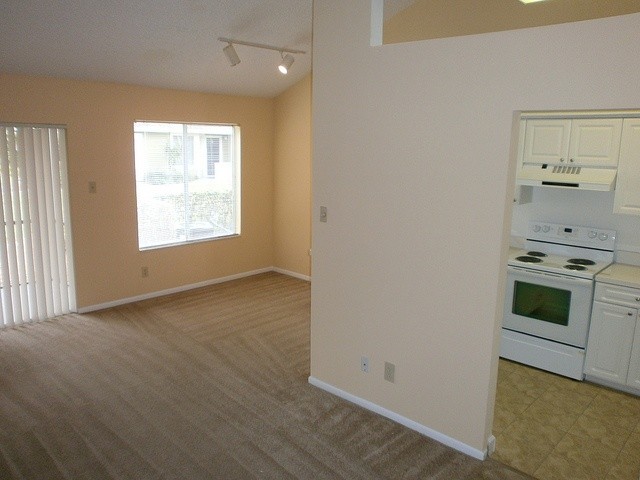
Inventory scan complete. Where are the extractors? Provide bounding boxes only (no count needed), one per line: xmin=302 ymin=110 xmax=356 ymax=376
xmin=516 ymin=165 xmax=618 ymax=193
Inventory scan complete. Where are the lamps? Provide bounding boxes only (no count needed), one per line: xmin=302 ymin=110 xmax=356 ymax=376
xmin=215 ymin=34 xmax=310 ymax=76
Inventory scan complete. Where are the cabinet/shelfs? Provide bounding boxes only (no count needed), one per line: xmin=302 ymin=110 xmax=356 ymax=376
xmin=524 ymin=118 xmax=622 ymax=167
xmin=614 ymin=114 xmax=640 ymax=213
xmin=583 ymin=282 xmax=640 ymax=398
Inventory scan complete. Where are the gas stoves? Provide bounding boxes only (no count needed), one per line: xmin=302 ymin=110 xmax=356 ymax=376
xmin=507 ymin=250 xmax=617 ymax=280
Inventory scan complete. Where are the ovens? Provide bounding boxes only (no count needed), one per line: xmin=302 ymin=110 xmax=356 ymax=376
xmin=501 ymin=264 xmax=594 ymax=349
